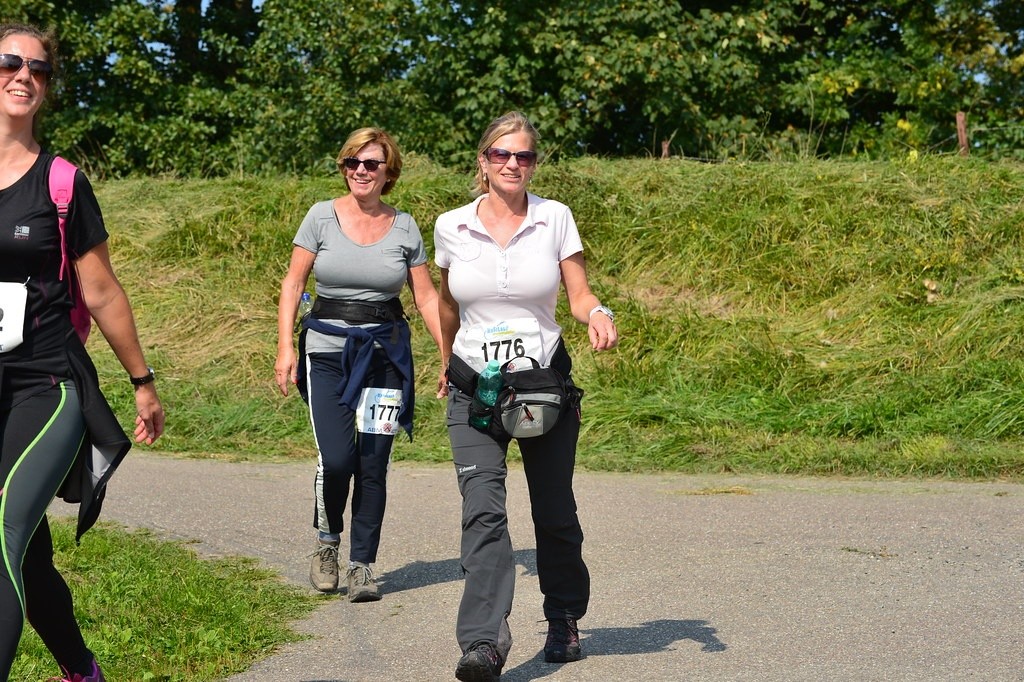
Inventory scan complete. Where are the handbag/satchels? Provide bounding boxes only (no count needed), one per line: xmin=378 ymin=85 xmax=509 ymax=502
xmin=487 ymin=367 xmax=567 ymax=438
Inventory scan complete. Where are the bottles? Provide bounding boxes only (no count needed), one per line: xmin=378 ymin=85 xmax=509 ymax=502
xmin=471 ymin=360 xmax=499 ymax=428
xmin=301 ymin=293 xmax=312 ymax=314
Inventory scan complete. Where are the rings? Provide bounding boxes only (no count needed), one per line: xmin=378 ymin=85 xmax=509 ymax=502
xmin=277 ymin=373 xmax=281 ymax=376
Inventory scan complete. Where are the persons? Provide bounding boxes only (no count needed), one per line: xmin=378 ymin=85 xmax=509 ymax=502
xmin=273 ymin=126 xmax=444 ymax=604
xmin=0 ymin=19 xmax=165 ymax=682
xmin=435 ymin=110 xmax=619 ymax=682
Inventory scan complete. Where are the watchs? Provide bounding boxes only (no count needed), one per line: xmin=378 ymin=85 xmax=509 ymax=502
xmin=130 ymin=367 xmax=155 ymax=385
xmin=590 ymin=306 xmax=614 ymax=320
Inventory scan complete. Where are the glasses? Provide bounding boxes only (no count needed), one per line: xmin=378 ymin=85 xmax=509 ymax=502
xmin=483 ymin=146 xmax=538 ymax=167
xmin=344 ymin=156 xmax=387 ymax=172
xmin=0 ymin=53 xmax=54 ymax=88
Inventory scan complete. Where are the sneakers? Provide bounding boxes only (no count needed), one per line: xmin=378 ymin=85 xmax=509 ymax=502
xmin=345 ymin=558 xmax=382 ymax=602
xmin=44 ymin=656 xmax=107 ymax=682
xmin=305 ymin=531 xmax=343 ymax=593
xmin=454 ymin=641 xmax=504 ymax=682
xmin=537 ymin=617 xmax=582 ymax=663
